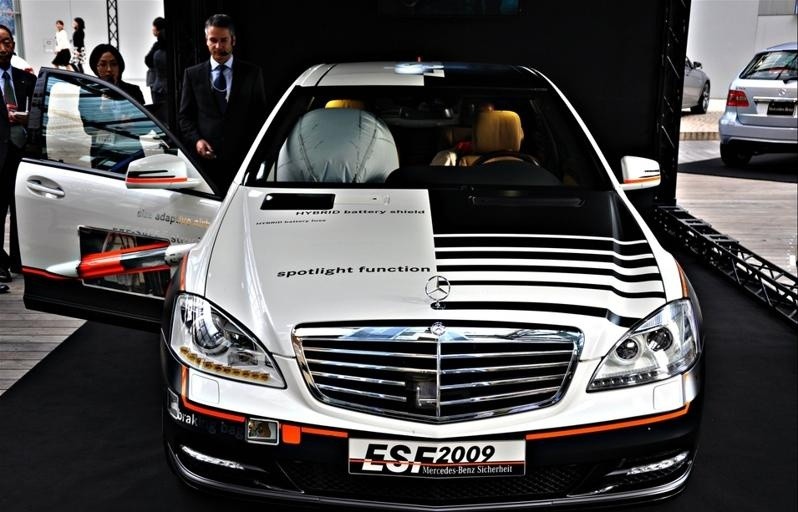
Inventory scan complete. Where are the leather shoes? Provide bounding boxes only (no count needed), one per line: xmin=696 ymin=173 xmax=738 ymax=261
xmin=10 ymin=263 xmax=23 ymax=274
xmin=0 ymin=284 xmax=10 ymax=294
xmin=0 ymin=271 xmax=12 ymax=283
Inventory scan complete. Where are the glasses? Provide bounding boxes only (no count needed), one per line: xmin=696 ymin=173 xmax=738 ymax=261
xmin=97 ymin=60 xmax=118 ymax=69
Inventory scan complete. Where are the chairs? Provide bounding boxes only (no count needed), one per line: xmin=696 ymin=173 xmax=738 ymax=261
xmin=459 ymin=110 xmax=534 ymax=167
xmin=447 ymin=126 xmax=473 ymax=150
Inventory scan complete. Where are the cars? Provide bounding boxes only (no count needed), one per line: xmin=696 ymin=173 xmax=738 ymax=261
xmin=681 ymin=55 xmax=710 ymax=115
xmin=718 ymin=42 xmax=797 ymax=166
xmin=13 ymin=61 xmax=707 ymax=512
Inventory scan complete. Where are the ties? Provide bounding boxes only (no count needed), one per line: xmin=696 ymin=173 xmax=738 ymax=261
xmin=213 ymin=64 xmax=227 ymax=100
xmin=1 ymin=71 xmax=17 ymax=113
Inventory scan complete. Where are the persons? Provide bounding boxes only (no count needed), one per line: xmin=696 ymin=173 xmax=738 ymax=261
xmin=178 ymin=12 xmax=269 ymax=195
xmin=50 ymin=18 xmax=73 ymax=73
xmin=0 ymin=21 xmax=40 ymax=282
xmin=78 ymin=42 xmax=153 ymax=173
xmin=68 ymin=17 xmax=87 ymax=75
xmin=151 ymin=31 xmax=170 ymax=127
xmin=143 ymin=16 xmax=166 ymax=141
